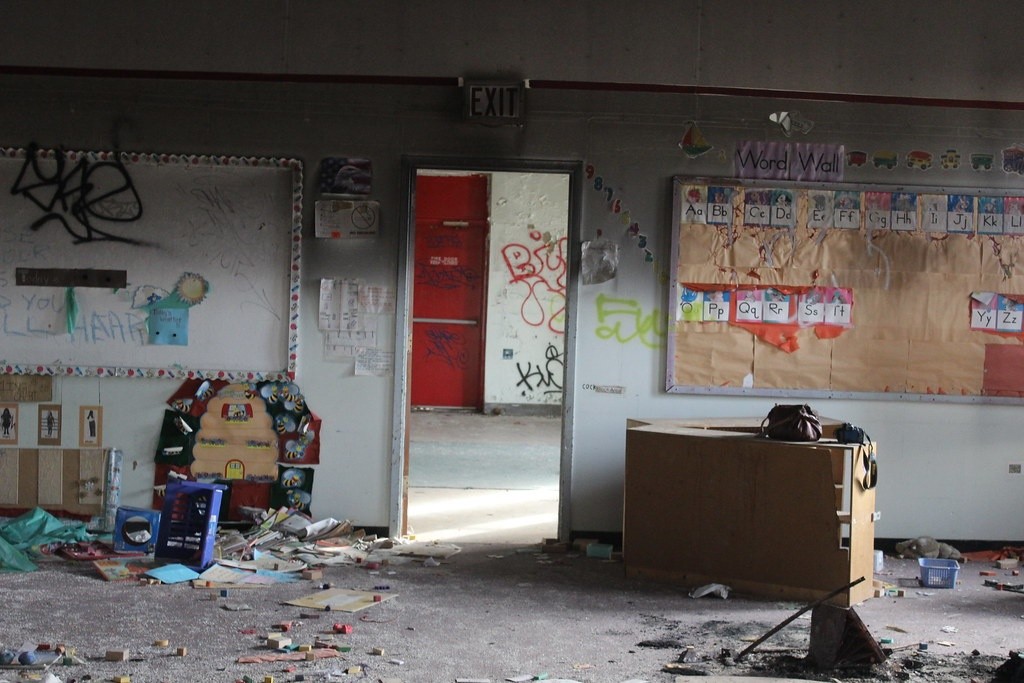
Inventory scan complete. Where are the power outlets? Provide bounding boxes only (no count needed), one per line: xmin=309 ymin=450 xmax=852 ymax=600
xmin=1009 ymin=464 xmax=1021 ymax=473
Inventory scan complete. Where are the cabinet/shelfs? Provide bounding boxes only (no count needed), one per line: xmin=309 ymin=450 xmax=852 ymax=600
xmin=622 ymin=415 xmax=879 ymax=609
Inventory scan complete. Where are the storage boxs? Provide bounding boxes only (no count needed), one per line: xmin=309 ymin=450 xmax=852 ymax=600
xmin=111 ymin=503 xmax=161 ymax=554
xmin=154 ymin=478 xmax=229 ymax=569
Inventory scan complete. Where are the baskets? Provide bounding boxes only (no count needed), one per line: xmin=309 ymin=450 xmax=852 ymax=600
xmin=918 ymin=558 xmax=960 ymax=589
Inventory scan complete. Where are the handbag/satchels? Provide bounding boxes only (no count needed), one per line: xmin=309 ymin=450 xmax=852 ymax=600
xmin=757 ymin=403 xmax=822 ymax=441
